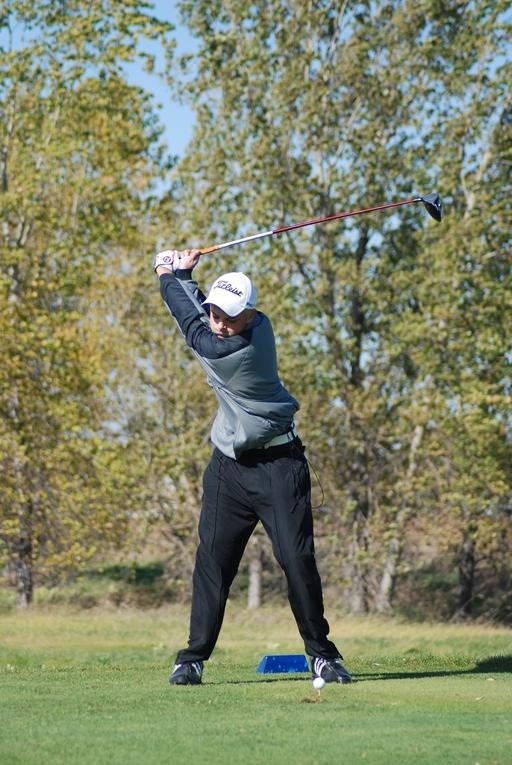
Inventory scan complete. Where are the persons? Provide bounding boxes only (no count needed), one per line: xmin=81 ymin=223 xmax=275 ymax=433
xmin=154 ymin=249 xmax=352 ymax=686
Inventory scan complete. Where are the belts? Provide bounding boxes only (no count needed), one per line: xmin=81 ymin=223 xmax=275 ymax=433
xmin=258 ymin=426 xmax=298 ymax=450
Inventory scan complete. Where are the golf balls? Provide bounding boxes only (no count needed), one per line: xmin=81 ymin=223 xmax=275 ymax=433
xmin=313 ymin=676 xmax=324 ymax=689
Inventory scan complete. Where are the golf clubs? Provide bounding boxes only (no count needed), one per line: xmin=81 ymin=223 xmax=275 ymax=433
xmin=199 ymin=193 xmax=442 ymax=255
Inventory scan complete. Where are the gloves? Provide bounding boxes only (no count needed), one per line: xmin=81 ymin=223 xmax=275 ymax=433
xmin=153 ymin=249 xmax=189 ymax=272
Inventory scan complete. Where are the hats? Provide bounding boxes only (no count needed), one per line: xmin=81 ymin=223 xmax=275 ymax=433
xmin=201 ymin=272 xmax=257 ymax=317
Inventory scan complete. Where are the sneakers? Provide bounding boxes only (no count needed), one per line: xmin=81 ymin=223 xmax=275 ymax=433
xmin=311 ymin=655 xmax=351 ymax=683
xmin=169 ymin=660 xmax=203 ymax=684
